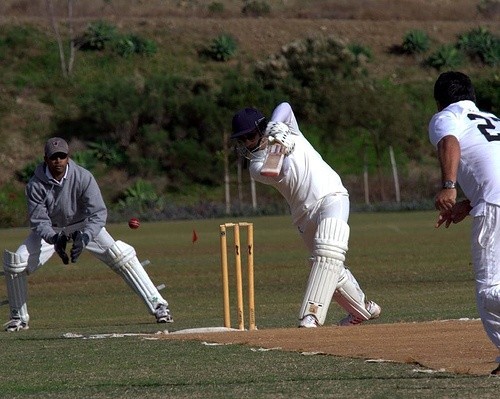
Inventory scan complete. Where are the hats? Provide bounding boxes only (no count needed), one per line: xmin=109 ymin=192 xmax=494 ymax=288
xmin=45 ymin=137 xmax=69 ymax=158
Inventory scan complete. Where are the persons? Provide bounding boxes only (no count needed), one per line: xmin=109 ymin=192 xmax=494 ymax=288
xmin=3 ymin=138 xmax=173 ymax=332
xmin=229 ymin=101 xmax=382 ymax=327
xmin=428 ymin=72 xmax=500 ymax=374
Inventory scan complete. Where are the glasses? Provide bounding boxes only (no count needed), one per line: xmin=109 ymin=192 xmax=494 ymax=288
xmin=49 ymin=152 xmax=67 ymax=161
xmin=236 ymin=128 xmax=264 ymax=140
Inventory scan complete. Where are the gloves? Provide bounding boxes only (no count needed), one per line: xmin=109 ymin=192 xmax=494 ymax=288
xmin=51 ymin=230 xmax=69 ymax=265
xmin=70 ymin=231 xmax=89 ymax=263
xmin=263 ymin=121 xmax=296 ymax=157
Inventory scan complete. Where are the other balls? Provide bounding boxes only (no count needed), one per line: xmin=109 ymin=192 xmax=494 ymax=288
xmin=128 ymin=217 xmax=141 ymax=230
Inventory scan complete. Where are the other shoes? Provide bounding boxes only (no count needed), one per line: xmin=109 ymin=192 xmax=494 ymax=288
xmin=3 ymin=318 xmax=29 ymax=332
xmin=297 ymin=314 xmax=318 ymax=328
xmin=337 ymin=301 xmax=381 ymax=325
xmin=491 ymin=363 xmax=500 ymax=374
xmin=155 ymin=304 xmax=173 ymax=322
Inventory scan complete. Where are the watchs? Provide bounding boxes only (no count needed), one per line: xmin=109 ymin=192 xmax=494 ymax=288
xmin=441 ymin=180 xmax=457 ymax=189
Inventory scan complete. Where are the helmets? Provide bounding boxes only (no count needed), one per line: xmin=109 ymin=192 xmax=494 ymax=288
xmin=229 ymin=107 xmax=267 ymax=160
